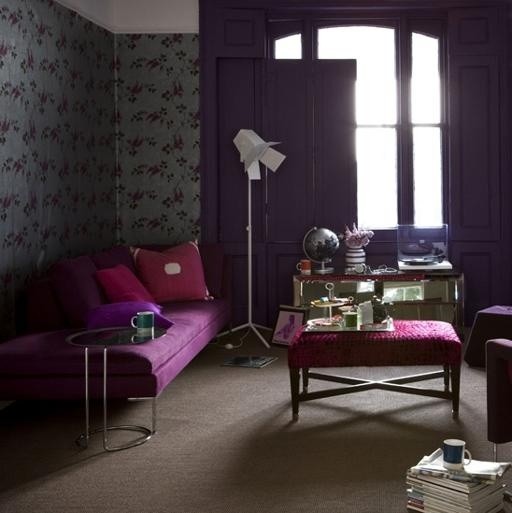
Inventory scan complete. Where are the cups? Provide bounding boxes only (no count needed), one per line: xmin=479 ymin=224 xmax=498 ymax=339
xmin=296 ymin=258 xmax=312 ymax=275
xmin=132 ymin=310 xmax=155 ymax=337
xmin=442 ymin=438 xmax=471 ymax=471
xmin=342 ymin=311 xmax=358 ymax=328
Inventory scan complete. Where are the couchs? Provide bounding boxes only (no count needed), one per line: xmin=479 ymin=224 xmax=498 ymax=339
xmin=1 ymin=239 xmax=231 ymax=439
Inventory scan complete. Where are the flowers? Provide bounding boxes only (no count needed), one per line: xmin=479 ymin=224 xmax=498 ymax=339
xmin=341 ymin=222 xmax=375 ymax=248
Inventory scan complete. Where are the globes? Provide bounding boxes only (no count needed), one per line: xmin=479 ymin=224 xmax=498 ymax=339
xmin=303 ymin=227 xmax=340 ymax=274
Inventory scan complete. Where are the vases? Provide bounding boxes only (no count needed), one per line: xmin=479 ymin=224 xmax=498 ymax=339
xmin=343 ymin=246 xmax=367 ymax=270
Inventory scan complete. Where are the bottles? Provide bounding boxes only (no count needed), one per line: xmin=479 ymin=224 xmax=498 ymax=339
xmin=357 ymin=301 xmax=374 ymax=325
xmin=371 ymin=295 xmax=388 ymax=323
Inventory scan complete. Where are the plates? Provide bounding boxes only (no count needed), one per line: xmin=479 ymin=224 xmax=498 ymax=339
xmin=307 ymin=317 xmax=334 ymax=329
xmin=311 ymin=297 xmax=349 ymax=308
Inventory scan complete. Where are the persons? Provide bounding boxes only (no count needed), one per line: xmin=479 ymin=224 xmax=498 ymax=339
xmin=274 ymin=315 xmax=299 ymax=341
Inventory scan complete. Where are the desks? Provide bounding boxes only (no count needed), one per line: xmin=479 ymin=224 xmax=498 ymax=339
xmin=292 ymin=268 xmax=465 ymax=346
xmin=67 ymin=327 xmax=167 ymax=452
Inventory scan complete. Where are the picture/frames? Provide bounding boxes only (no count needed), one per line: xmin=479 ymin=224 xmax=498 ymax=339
xmin=270 ymin=302 xmax=310 ymax=351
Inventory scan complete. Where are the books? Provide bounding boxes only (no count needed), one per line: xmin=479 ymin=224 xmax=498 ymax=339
xmin=405 ymin=449 xmax=512 ymax=512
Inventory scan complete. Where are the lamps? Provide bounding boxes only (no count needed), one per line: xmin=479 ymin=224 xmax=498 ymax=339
xmin=208 ymin=126 xmax=287 ymax=370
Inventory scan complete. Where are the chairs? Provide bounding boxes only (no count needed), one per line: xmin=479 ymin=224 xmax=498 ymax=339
xmin=461 ymin=304 xmax=512 ymax=369
xmin=486 ymin=338 xmax=511 ymax=464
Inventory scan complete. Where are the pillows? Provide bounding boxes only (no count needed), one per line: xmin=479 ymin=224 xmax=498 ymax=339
xmin=52 ymin=235 xmax=215 ymax=327
